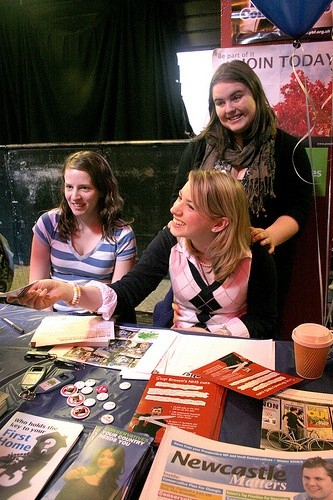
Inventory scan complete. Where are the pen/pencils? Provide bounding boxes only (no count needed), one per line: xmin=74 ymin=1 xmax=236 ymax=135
xmin=0 ymin=315 xmax=24 ymax=334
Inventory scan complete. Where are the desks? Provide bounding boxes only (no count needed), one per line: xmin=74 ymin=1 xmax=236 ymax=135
xmin=0 ymin=302 xmax=333 ymax=500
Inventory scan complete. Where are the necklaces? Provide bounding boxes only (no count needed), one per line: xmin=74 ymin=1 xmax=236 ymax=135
xmin=198 ymin=261 xmax=212 ymax=267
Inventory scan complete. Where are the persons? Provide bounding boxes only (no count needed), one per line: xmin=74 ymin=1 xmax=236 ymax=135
xmin=54 ymin=445 xmax=125 ymax=500
xmin=6 ymin=170 xmax=286 ymax=339
xmin=294 ymin=456 xmax=333 ymax=500
xmin=170 ymin=60 xmax=314 ymax=330
xmin=131 ymin=406 xmax=166 ymax=439
xmin=281 ymin=405 xmax=306 ymax=444
xmin=218 ymin=352 xmax=253 ymax=372
xmin=30 ymin=151 xmax=137 ymax=318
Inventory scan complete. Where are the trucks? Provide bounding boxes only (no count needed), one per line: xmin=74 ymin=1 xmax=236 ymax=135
xmin=234 ymin=1 xmax=333 ymax=45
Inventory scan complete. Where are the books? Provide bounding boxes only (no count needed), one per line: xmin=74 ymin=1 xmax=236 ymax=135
xmin=0 ymin=314 xmax=333 ymax=500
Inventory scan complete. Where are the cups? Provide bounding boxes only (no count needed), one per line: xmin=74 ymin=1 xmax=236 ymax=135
xmin=292 ymin=323 xmax=333 ymax=379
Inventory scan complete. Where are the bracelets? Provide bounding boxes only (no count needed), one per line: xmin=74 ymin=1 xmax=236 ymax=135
xmin=217 ymin=329 xmax=228 ymax=335
xmin=68 ymin=282 xmax=80 ymax=308
